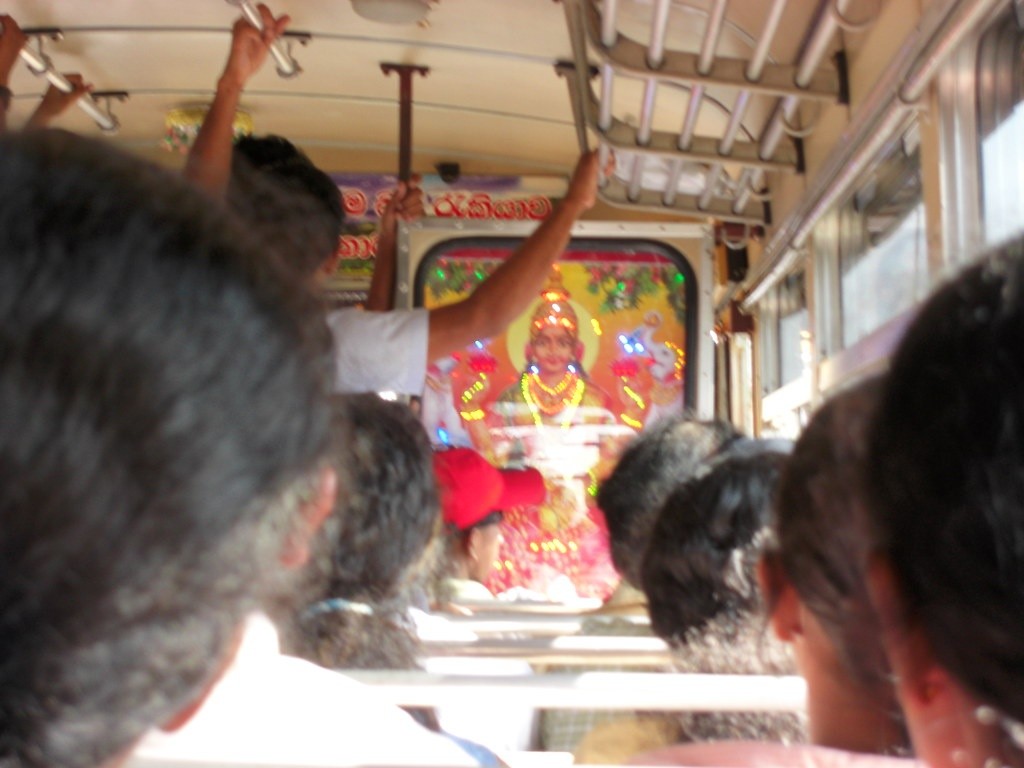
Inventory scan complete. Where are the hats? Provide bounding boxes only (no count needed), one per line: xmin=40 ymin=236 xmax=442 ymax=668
xmin=427 ymin=449 xmax=547 ymax=532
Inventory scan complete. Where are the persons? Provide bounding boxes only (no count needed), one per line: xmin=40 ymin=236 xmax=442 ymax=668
xmin=0 ymin=0 xmax=1023 ymax=768
